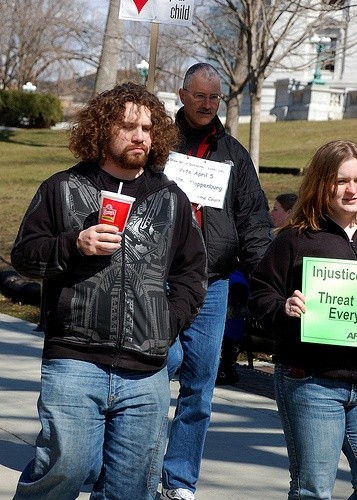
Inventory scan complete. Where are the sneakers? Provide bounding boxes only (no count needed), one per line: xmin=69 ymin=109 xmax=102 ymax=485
xmin=160 ymin=486 xmax=196 ymax=500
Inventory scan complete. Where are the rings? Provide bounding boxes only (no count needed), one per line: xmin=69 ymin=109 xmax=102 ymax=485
xmin=290 ymin=304 xmax=292 ymax=312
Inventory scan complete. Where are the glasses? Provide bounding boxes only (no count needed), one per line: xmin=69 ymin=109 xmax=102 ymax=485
xmin=183 ymin=89 xmax=223 ymax=103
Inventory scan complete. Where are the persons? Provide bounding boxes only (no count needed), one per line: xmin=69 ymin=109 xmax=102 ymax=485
xmin=10 ymin=83 xmax=207 ymax=500
xmin=245 ymin=140 xmax=357 ymax=500
xmin=166 ymin=63 xmax=297 ymax=500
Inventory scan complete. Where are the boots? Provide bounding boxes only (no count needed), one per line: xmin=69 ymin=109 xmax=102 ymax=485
xmin=216 ymin=337 xmax=240 ymax=384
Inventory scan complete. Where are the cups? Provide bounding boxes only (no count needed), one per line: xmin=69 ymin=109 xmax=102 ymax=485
xmin=98 ymin=190 xmax=136 ymax=234
